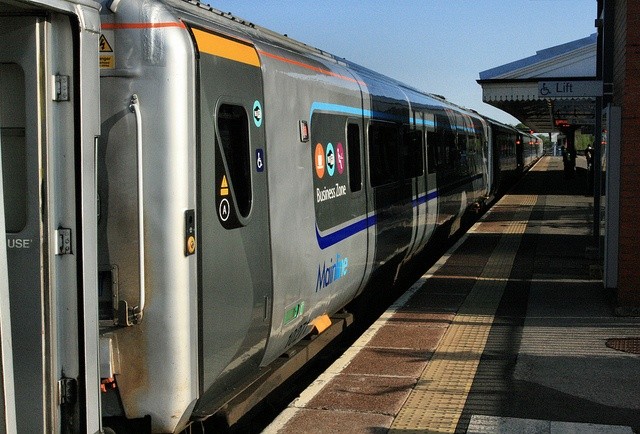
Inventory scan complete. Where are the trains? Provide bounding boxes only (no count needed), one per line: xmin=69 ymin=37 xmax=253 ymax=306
xmin=0 ymin=0 xmax=543 ymax=434
